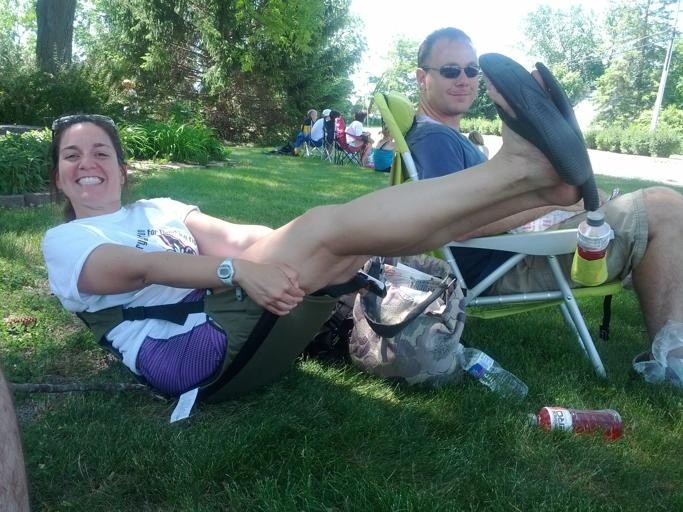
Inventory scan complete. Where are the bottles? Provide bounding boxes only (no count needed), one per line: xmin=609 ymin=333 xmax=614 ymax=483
xmin=527 ymin=406 xmax=622 ymax=441
xmin=574 ymin=211 xmax=610 ymax=287
xmin=453 ymin=344 xmax=527 ymax=405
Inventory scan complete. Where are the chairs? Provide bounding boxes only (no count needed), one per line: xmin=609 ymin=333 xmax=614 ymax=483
xmin=374 ymin=92 xmax=624 ymax=377
xmin=300 ymin=117 xmax=367 ymax=167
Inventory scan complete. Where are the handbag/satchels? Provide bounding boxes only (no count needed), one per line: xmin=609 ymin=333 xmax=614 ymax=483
xmin=302 ymin=288 xmax=353 ymax=365
xmin=349 ymin=255 xmax=467 ymax=386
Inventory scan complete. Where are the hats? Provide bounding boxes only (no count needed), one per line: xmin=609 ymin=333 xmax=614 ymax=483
xmin=322 ymin=109 xmax=332 ymax=117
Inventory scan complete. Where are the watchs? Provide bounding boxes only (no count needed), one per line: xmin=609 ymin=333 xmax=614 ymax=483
xmin=216 ymin=257 xmax=235 ymax=287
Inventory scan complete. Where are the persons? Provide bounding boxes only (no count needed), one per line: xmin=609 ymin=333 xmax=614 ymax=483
xmin=301 ymin=108 xmax=375 ymax=167
xmin=39 ymin=52 xmax=600 ymax=403
xmin=402 ymin=25 xmax=683 ymax=384
xmin=373 ymin=124 xmax=396 ymax=173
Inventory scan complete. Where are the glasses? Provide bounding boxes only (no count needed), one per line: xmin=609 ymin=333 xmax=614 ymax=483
xmin=52 ymin=114 xmax=114 ymax=130
xmin=424 ymin=63 xmax=480 ymax=78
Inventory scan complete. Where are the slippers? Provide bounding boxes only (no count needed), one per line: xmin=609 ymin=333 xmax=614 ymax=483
xmin=476 ymin=52 xmax=600 ymax=211
